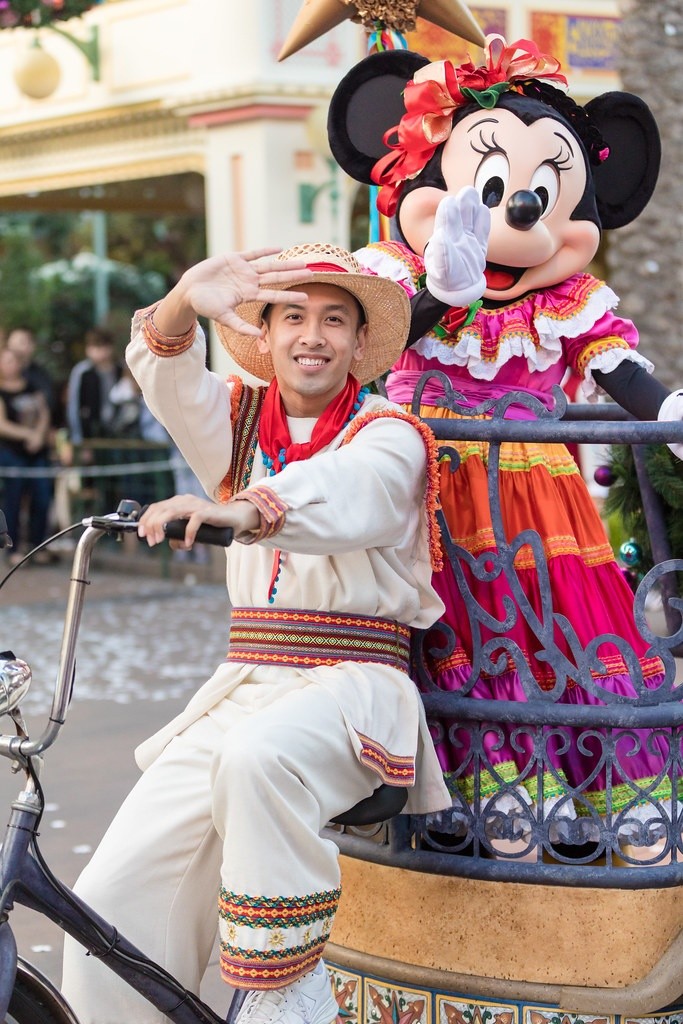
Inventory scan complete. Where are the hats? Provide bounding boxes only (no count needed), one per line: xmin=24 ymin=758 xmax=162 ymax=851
xmin=215 ymin=243 xmax=412 ymax=386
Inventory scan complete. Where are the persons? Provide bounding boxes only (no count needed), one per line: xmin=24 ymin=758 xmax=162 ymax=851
xmin=2 ymin=324 xmax=208 ymax=565
xmin=60 ymin=242 xmax=453 ymax=1024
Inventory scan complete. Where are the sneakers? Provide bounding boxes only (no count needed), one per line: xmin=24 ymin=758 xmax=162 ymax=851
xmin=233 ymin=956 xmax=339 ymax=1024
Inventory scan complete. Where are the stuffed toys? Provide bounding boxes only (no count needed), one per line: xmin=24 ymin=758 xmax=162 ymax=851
xmin=325 ymin=29 xmax=683 ymax=871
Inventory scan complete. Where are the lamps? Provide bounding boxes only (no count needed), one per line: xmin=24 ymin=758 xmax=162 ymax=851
xmin=301 ymin=101 xmax=338 ymax=224
xmin=16 ymin=4 xmax=100 ymax=98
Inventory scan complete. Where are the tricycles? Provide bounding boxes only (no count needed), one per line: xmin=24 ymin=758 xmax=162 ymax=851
xmin=1 ymin=391 xmax=683 ymax=1024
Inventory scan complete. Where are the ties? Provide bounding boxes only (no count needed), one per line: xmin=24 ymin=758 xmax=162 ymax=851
xmin=259 ymin=372 xmax=371 ymax=604
